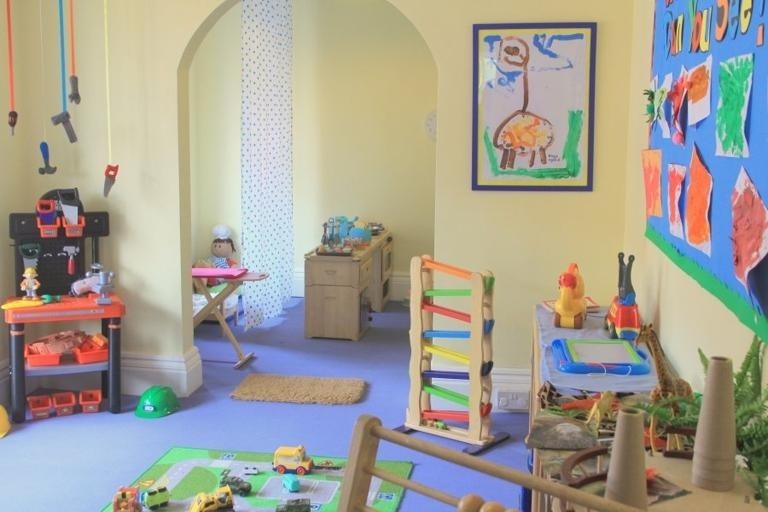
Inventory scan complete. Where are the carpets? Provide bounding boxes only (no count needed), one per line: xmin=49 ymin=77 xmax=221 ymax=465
xmin=99 ymin=447 xmax=412 ymax=512
xmin=228 ymin=373 xmax=366 ymax=404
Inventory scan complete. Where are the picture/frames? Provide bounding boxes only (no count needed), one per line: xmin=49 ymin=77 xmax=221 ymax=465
xmin=471 ymin=22 xmax=598 ymax=191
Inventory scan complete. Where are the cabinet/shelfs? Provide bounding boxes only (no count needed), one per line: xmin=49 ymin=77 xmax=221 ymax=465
xmin=0 ymin=212 xmax=126 ymax=422
xmin=303 ymin=229 xmax=394 ymax=341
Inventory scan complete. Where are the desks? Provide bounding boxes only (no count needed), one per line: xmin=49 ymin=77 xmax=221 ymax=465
xmin=192 ymin=271 xmax=270 ymax=369
xmin=528 ymin=300 xmax=768 ymax=512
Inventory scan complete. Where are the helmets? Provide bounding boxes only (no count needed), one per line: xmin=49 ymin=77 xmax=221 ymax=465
xmin=135 ymin=384 xmax=181 ymax=419
xmin=21 ymin=267 xmax=39 ymax=278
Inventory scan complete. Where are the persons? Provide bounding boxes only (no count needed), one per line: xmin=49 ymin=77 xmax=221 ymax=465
xmin=201 ymin=223 xmax=240 ymax=285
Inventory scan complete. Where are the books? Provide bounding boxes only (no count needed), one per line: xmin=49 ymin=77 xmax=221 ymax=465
xmin=542 ymin=295 xmax=600 ymax=314
